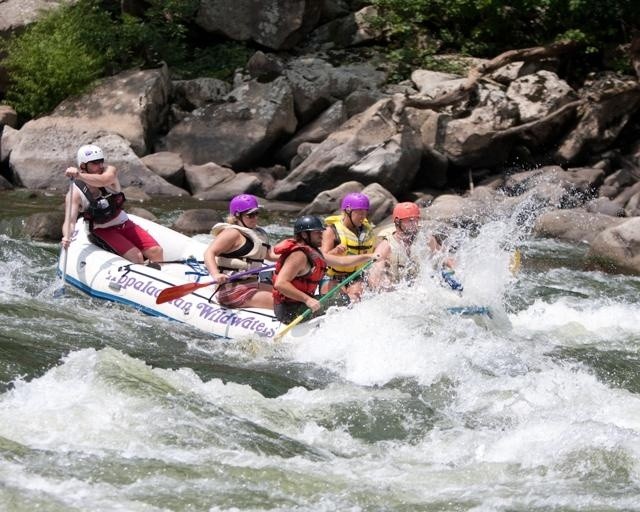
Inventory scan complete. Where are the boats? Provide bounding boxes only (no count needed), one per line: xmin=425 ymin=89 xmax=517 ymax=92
xmin=58 ymin=183 xmax=511 ymax=349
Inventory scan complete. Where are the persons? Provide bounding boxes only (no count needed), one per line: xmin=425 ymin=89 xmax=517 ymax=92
xmin=369 ymin=203 xmax=453 ymax=294
xmin=61 ymin=145 xmax=164 ymax=267
xmin=437 ymin=219 xmax=520 ymax=315
xmin=322 ymin=193 xmax=378 ymax=301
xmin=205 ymin=194 xmax=274 ymax=309
xmin=268 ymin=216 xmax=382 ymax=325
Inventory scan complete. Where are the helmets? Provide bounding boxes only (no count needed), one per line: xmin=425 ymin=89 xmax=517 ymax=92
xmin=392 ymin=202 xmax=420 ymax=221
xmin=341 ymin=193 xmax=369 ymax=210
xmin=230 ymin=195 xmax=263 ymax=216
xmin=294 ymin=215 xmax=326 ymax=233
xmin=78 ymin=144 xmax=104 ymax=167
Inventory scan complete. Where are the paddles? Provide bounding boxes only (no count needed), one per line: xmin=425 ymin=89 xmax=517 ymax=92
xmin=55 ymin=175 xmax=74 ymax=299
xmin=156 ymin=264 xmax=276 ymax=304
xmin=248 ymin=256 xmax=380 ymax=355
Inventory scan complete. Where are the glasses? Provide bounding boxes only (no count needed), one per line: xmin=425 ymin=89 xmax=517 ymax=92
xmin=245 ymin=212 xmax=258 ymax=218
